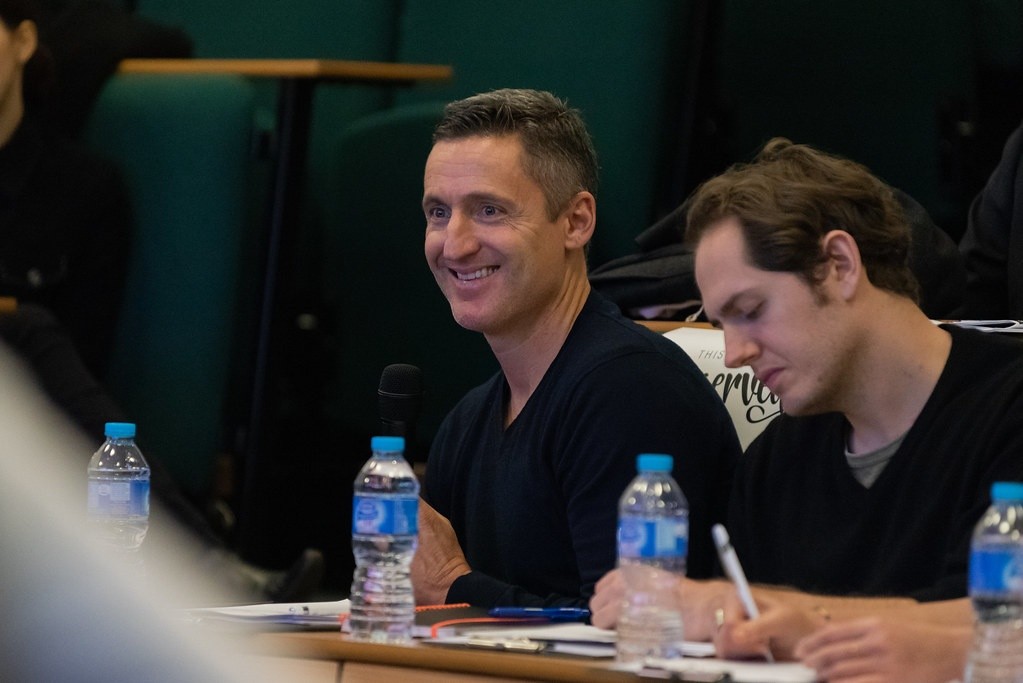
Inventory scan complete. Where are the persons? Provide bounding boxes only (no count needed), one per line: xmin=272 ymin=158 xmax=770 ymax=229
xmin=588 ymin=135 xmax=1023 ymax=637
xmin=709 ymin=585 xmax=974 ymax=682
xmin=957 ymin=125 xmax=1022 ymax=319
xmin=408 ymin=86 xmax=741 ymax=607
xmin=0 ymin=0 xmax=130 ymax=379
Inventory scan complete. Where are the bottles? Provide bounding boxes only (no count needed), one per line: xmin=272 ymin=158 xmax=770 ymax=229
xmin=345 ymin=437 xmax=419 ymax=645
xmin=89 ymin=424 xmax=151 ymax=562
xmin=962 ymin=483 xmax=1022 ymax=683
xmin=616 ymin=454 xmax=688 ymax=672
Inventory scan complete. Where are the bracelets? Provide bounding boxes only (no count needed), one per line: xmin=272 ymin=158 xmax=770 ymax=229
xmin=814 ymin=606 xmax=833 ymax=621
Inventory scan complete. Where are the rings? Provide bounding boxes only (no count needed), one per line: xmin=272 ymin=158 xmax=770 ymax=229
xmin=714 ymin=609 xmax=725 ymax=629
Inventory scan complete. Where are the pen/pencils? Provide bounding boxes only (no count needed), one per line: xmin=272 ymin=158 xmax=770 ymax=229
xmin=712 ymin=523 xmax=776 ymax=664
xmin=487 ymin=605 xmax=590 ymax=619
